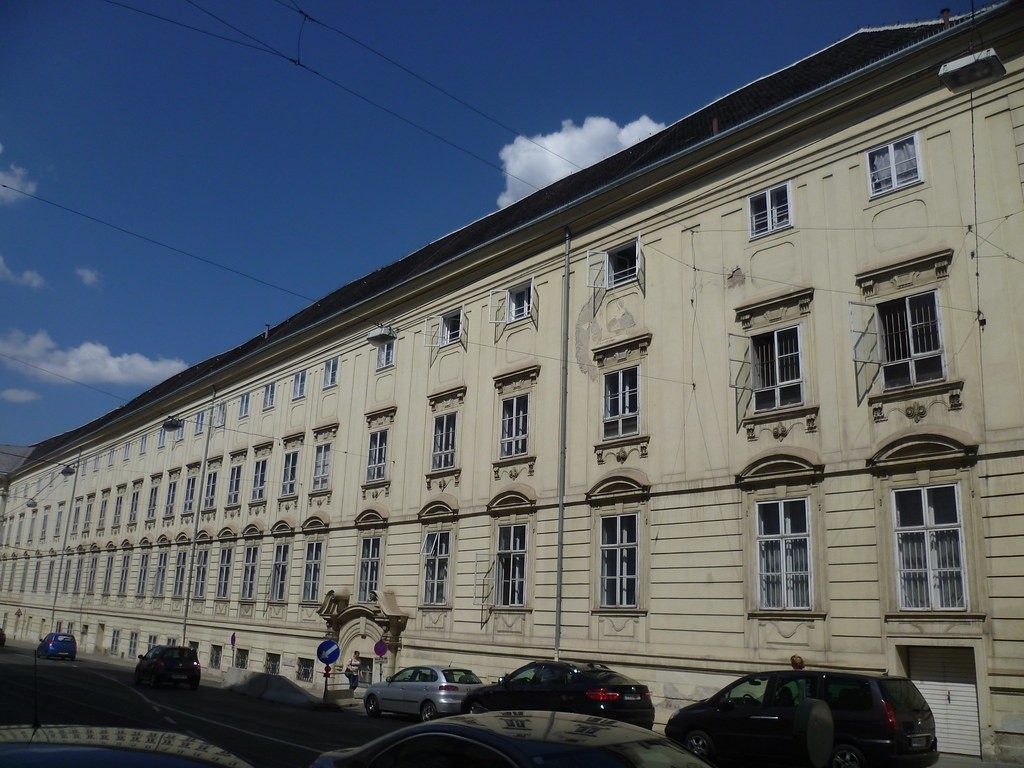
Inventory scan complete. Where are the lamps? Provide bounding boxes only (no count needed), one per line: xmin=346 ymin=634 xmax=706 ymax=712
xmin=162 ymin=419 xmax=183 ymax=432
xmin=938 ymin=47 xmax=1007 ymax=95
xmin=366 ymin=326 xmax=398 ymax=347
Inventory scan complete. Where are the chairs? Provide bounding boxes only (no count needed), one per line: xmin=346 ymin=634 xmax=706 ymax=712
xmin=777 ymin=687 xmax=795 ymax=706
xmin=416 ymin=673 xmax=430 ymax=682
xmin=459 ymin=675 xmax=467 ymax=683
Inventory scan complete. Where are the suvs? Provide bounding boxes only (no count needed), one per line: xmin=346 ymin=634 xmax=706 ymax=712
xmin=664 ymin=668 xmax=941 ymax=767
xmin=462 ymin=657 xmax=655 ymax=732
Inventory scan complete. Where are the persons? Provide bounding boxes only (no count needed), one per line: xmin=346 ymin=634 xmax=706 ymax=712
xmin=346 ymin=650 xmax=361 ymax=693
xmin=791 ymin=655 xmax=805 ymax=670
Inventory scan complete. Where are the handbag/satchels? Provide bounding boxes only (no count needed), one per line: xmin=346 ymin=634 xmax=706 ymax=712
xmin=345 ymin=659 xmax=352 ymax=678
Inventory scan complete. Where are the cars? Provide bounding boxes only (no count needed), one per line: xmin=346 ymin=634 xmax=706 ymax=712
xmin=0 ymin=628 xmax=7 ymax=647
xmin=363 ymin=663 xmax=484 ymax=724
xmin=0 ymin=724 xmax=259 ymax=768
xmin=134 ymin=644 xmax=201 ymax=691
xmin=37 ymin=632 xmax=77 ymax=661
xmin=308 ymin=709 xmax=717 ymax=768
